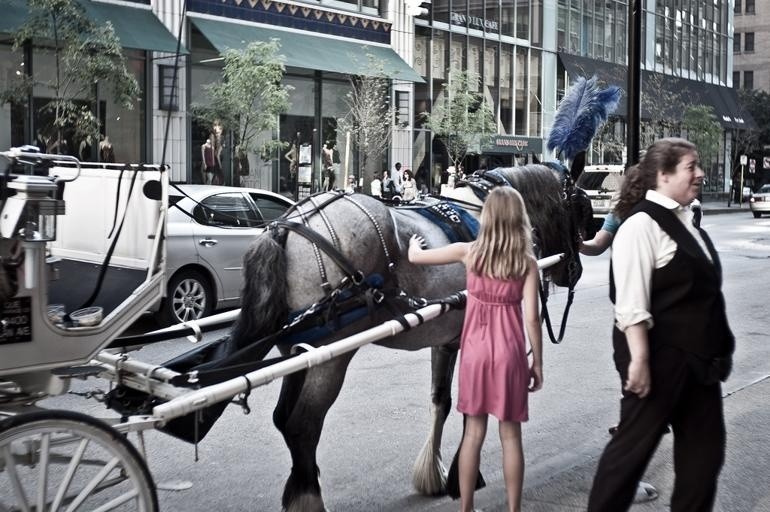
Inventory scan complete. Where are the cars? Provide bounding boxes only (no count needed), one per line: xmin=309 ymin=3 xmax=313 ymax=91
xmin=157 ymin=179 xmax=313 ymax=326
xmin=750 ymin=184 xmax=770 ymax=217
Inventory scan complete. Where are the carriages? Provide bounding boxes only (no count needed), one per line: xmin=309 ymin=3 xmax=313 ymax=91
xmin=0 ymin=142 xmax=586 ymax=512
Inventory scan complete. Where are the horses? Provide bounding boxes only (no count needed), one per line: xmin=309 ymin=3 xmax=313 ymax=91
xmin=222 ymin=150 xmax=595 ymax=512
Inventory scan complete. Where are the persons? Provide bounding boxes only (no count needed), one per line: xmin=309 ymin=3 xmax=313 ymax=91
xmin=407 ymin=185 xmax=544 ymax=512
xmin=446 ymin=165 xmax=457 ymax=189
xmin=578 ymin=209 xmax=659 ymax=506
xmin=201 ymin=118 xmax=337 ymax=195
xmin=584 ymin=138 xmax=736 ymax=512
xmin=34 ymin=132 xmax=118 ymax=170
xmin=346 ymin=162 xmax=421 ymax=205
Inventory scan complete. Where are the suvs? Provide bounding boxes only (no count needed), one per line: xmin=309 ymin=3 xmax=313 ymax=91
xmin=573 ymin=163 xmax=703 ymax=231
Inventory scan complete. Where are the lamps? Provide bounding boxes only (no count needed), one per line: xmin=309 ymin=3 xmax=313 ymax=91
xmin=396 ymin=90 xmax=409 ymax=126
xmin=157 ymin=64 xmax=180 ymax=111
